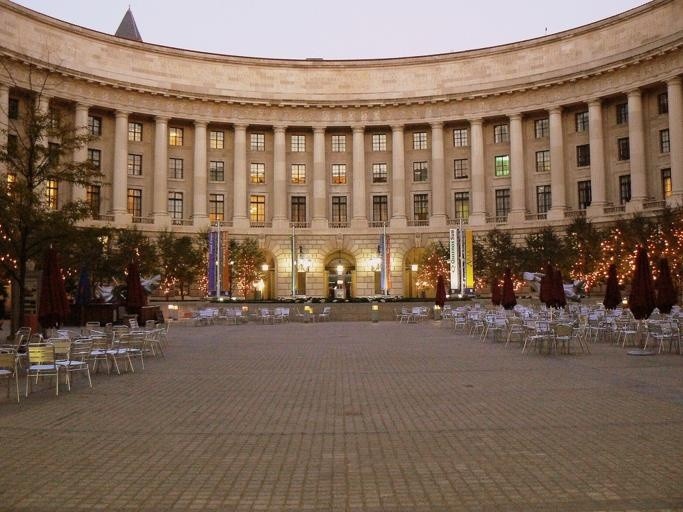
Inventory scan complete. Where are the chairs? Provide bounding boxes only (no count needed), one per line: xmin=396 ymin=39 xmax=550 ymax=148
xmin=392 ymin=299 xmax=683 ymax=357
xmin=178 ymin=304 xmax=332 ymax=327
xmin=0 ymin=312 xmax=171 ymax=406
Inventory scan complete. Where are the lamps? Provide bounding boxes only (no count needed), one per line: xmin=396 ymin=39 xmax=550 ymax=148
xmin=306 ymin=259 xmax=312 ymax=272
xmin=370 ymin=301 xmax=380 ymax=323
xmin=407 ymin=248 xmax=420 ymax=272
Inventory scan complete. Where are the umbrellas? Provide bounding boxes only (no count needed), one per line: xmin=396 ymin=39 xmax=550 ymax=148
xmin=602 ymin=267 xmax=621 ymax=309
xmin=37 ymin=248 xmax=71 ymax=339
xmin=502 ymin=279 xmax=516 ymax=309
xmin=654 ymin=259 xmax=676 ymax=315
xmin=492 ymin=285 xmax=499 ymax=305
xmin=539 ymin=263 xmax=553 ymax=308
xmin=435 ymin=276 xmax=445 ymax=306
xmin=125 ymin=263 xmax=145 ymax=314
xmin=550 ymin=270 xmax=567 ymax=309
xmin=627 ymin=248 xmax=654 ymax=319
